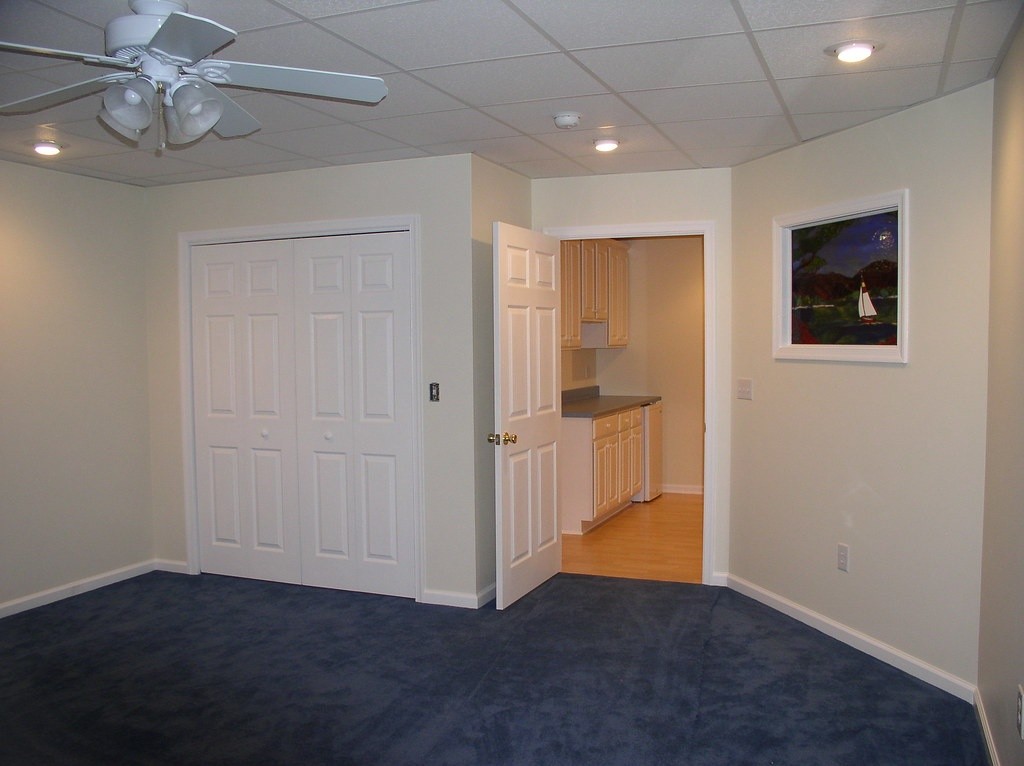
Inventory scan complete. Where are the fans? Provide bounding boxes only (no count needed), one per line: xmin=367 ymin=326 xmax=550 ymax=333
xmin=0 ymin=0 xmax=391 ymax=143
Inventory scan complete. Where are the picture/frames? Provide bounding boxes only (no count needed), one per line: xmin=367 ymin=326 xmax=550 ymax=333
xmin=772 ymin=186 xmax=911 ymax=364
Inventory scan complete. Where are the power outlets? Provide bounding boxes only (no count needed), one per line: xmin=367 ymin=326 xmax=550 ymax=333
xmin=1015 ymin=684 xmax=1024 ymax=741
xmin=837 ymin=543 xmax=850 ymax=573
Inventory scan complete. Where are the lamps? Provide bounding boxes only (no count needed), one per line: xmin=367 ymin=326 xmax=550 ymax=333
xmin=98 ymin=74 xmax=226 ymax=151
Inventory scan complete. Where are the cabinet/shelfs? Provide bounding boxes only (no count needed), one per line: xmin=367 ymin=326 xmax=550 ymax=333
xmin=560 ymin=240 xmax=582 ymax=350
xmin=582 ymin=240 xmax=630 ymax=349
xmin=559 ymin=406 xmax=645 ymax=535
xmin=582 ymin=239 xmax=609 ymax=321
